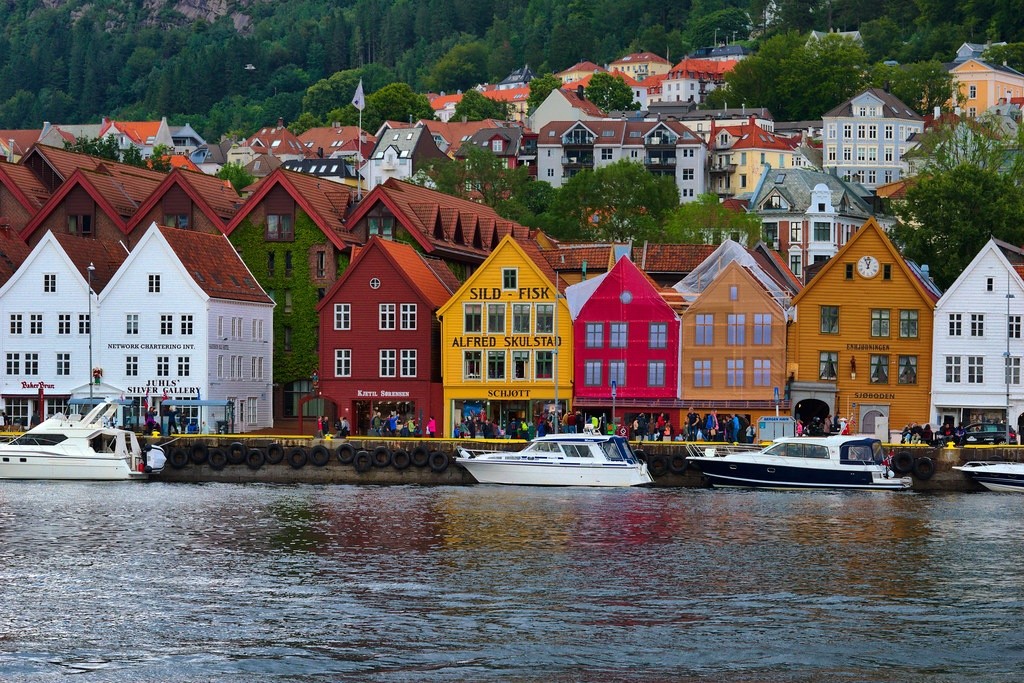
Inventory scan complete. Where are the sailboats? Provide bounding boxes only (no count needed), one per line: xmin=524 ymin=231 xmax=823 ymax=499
xmin=453 ymin=272 xmax=657 ymax=487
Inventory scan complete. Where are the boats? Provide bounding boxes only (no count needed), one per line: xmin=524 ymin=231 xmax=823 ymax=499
xmin=0 ymin=399 xmax=167 ymax=481
xmin=683 ymin=433 xmax=914 ymax=490
xmin=952 ymin=460 xmax=1024 ymax=493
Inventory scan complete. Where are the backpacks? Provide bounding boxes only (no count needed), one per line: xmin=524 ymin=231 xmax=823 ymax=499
xmin=521 ymin=422 xmax=528 ymax=430
xmin=145 ymin=413 xmax=148 ymax=421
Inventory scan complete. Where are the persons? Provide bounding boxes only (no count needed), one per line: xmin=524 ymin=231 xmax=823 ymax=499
xmin=318 ymin=408 xmax=435 ymax=438
xmin=627 ymin=407 xmax=756 ymax=444
xmin=2 ymin=411 xmax=40 ymax=432
xmin=796 ymin=412 xmax=851 ymax=437
xmin=454 ymin=411 xmax=604 ymax=439
xmin=901 ymin=421 xmax=980 ymax=447
xmin=145 ymin=405 xmax=210 ymax=435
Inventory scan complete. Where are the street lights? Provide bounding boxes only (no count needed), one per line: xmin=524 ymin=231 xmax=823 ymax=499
xmin=1006 ymin=294 xmax=1014 ymax=445
xmin=553 ymin=295 xmax=564 ymax=434
xmin=88 ymin=266 xmax=96 ymax=411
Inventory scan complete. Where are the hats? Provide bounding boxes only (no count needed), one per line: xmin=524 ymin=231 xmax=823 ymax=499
xmin=512 ymin=418 xmax=515 ymax=420
xmin=840 ymin=417 xmax=848 ymax=421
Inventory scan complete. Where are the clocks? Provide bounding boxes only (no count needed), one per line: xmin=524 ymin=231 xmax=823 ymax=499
xmin=856 ymin=254 xmax=882 ymax=280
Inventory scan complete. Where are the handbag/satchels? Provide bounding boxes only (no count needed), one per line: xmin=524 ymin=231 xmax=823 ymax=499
xmin=426 ymin=426 xmax=430 ymax=434
xmin=711 ymin=429 xmax=716 ymax=435
xmin=464 ymin=430 xmax=471 ymax=438
xmin=371 ymin=417 xmax=376 ymax=428
xmin=386 ymin=431 xmax=391 ymax=434
xmin=342 ymin=426 xmax=347 ymax=432
xmin=377 ymin=428 xmax=382 ymax=436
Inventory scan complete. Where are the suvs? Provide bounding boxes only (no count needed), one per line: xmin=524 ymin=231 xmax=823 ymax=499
xmin=932 ymin=422 xmax=1017 ymax=446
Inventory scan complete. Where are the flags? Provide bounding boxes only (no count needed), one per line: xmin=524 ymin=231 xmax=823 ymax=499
xmin=352 ymin=78 xmax=365 ymax=111
